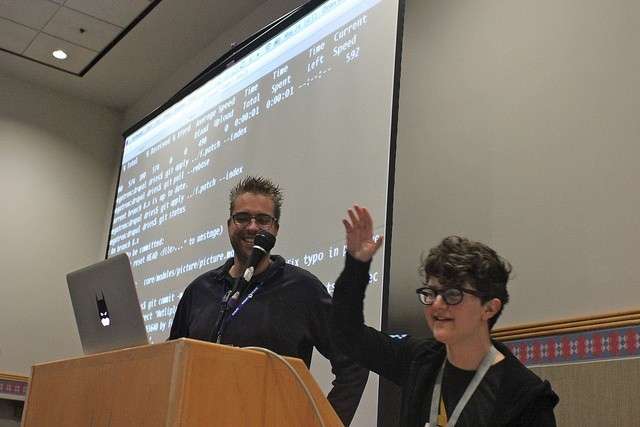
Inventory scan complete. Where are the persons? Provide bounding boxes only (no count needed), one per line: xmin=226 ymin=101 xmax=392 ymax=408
xmin=165 ymin=177 xmax=370 ymax=425
xmin=332 ymin=205 xmax=560 ymax=425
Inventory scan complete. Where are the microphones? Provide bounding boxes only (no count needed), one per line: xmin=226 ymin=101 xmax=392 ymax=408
xmin=224 ymin=230 xmax=276 ymax=313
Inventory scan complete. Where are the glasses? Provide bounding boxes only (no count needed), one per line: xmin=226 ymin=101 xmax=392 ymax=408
xmin=231 ymin=212 xmax=280 ymax=231
xmin=415 ymin=285 xmax=489 ymax=306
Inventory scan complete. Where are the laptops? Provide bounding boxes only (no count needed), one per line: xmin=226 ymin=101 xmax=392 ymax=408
xmin=66 ymin=254 xmax=151 ymax=355
